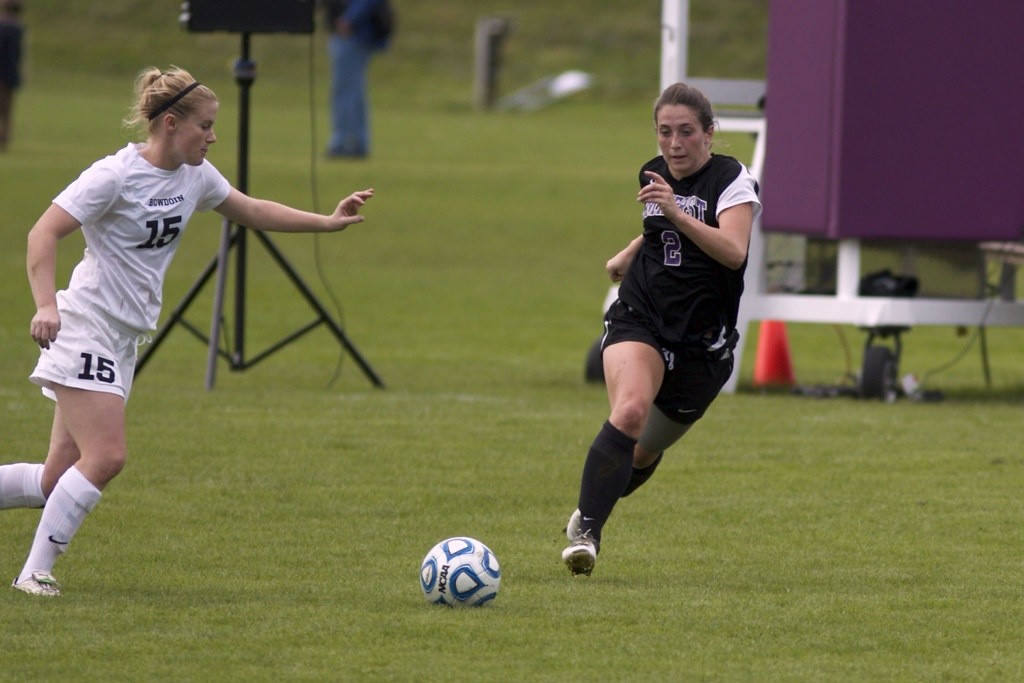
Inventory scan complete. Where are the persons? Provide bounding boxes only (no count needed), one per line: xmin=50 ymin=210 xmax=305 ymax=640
xmin=562 ymin=83 xmax=764 ymax=580
xmin=322 ymin=0 xmax=393 ymax=161
xmin=0 ymin=67 xmax=373 ymax=598
xmin=0 ymin=0 xmax=24 ymax=150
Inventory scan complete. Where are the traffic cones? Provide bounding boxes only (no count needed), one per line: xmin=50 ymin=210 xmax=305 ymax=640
xmin=738 ymin=320 xmax=801 ymax=394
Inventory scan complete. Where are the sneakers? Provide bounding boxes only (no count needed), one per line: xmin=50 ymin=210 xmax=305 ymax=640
xmin=567 ymin=508 xmax=582 ymax=541
xmin=562 ymin=529 xmax=600 ymax=576
xmin=11 ymin=572 xmax=61 ymax=597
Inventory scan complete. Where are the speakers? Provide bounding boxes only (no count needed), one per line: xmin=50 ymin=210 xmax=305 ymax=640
xmin=181 ymin=0 xmax=316 ymax=35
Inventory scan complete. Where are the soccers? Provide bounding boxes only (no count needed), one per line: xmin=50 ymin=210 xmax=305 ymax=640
xmin=418 ymin=535 xmax=502 ymax=608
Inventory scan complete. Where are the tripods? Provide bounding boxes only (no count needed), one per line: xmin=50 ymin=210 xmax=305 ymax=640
xmin=134 ymin=32 xmax=389 ymax=396
